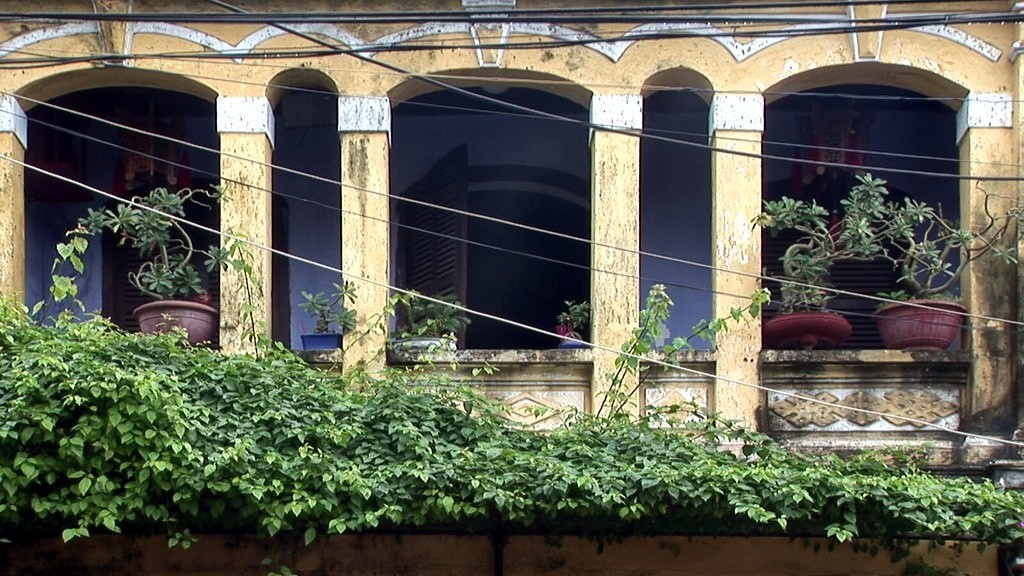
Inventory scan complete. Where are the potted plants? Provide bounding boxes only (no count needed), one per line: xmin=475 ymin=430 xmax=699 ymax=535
xmin=552 ymin=299 xmax=592 ymax=349
xmin=67 ymin=177 xmax=244 ymax=339
xmin=855 ymin=184 xmax=1024 ymax=352
xmin=753 ymin=168 xmax=901 ymax=347
xmin=298 ymin=278 xmax=365 ymax=350
xmin=386 ymin=285 xmax=469 ymax=351
